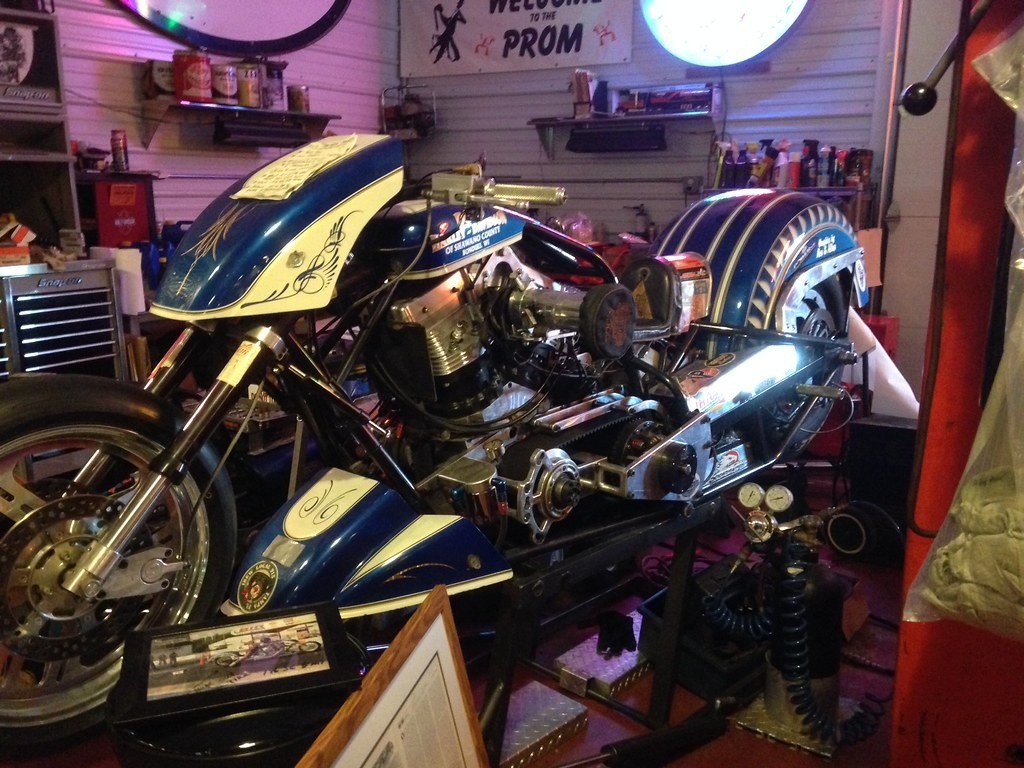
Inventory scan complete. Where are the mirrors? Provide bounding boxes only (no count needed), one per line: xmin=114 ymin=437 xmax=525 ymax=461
xmin=119 ymin=0 xmax=351 ymax=60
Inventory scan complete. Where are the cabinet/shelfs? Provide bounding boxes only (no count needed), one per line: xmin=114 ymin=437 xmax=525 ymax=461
xmin=0 ymin=7 xmax=87 ymax=258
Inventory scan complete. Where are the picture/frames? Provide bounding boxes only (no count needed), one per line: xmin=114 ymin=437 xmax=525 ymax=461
xmin=108 ymin=602 xmax=363 ymax=729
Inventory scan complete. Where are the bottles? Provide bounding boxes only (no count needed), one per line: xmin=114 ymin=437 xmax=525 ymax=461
xmin=635 ymin=204 xmax=648 ymax=237
xmin=721 ymin=144 xmax=874 ymax=186
xmin=172 ymin=50 xmax=311 ymax=111
xmin=647 ymin=222 xmax=656 ymax=243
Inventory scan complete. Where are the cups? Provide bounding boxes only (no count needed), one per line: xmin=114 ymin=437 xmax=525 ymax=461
xmin=846 ymin=173 xmax=861 ymax=186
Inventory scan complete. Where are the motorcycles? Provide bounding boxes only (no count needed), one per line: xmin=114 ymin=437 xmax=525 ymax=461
xmin=0 ymin=136 xmax=868 ymax=748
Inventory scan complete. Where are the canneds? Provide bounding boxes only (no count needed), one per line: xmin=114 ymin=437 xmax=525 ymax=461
xmin=110 ymin=129 xmax=130 ymax=171
xmin=173 ymin=50 xmax=311 ymax=114
